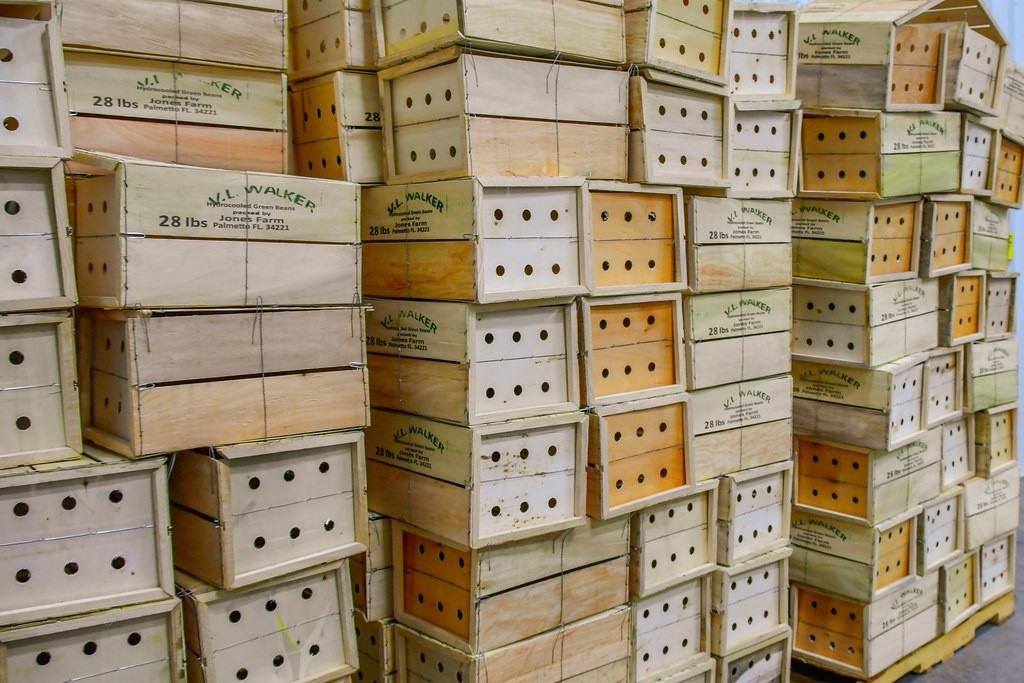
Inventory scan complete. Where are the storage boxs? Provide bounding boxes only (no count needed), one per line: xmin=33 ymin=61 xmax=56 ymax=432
xmin=72 ymin=148 xmax=362 ymax=312
xmin=55 ymin=0 xmax=288 ymax=69
xmin=787 ymin=571 xmax=941 ymax=680
xmin=711 ymin=547 xmax=794 ymax=659
xmin=0 ymin=443 xmax=175 ymax=626
xmin=174 ymin=558 xmax=359 ymax=683
xmin=63 ymin=46 xmax=289 ymax=175
xmin=288 ymin=0 xmax=380 ymax=83
xmin=167 ymin=430 xmax=370 ymax=592
xmin=394 ymin=605 xmax=634 ymax=683
xmin=629 ymin=572 xmax=711 ymax=683
xmin=0 ymin=0 xmax=75 ymax=161
xmin=288 ymin=70 xmax=386 ymax=188
xmin=76 ymin=307 xmax=370 ymax=461
xmin=0 ymin=596 xmax=188 ymax=683
xmin=389 ymin=519 xmax=632 ymax=656
xmin=713 ymin=629 xmax=793 ymax=683
xmin=0 ymin=155 xmax=80 ymax=314
xmin=363 ymin=0 xmax=1024 ymax=634
xmin=0 ymin=309 xmax=84 ymax=471
